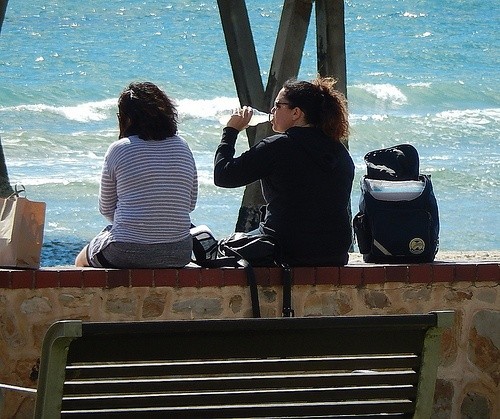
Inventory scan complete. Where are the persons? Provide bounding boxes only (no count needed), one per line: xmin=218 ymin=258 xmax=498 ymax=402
xmin=75 ymin=80 xmax=198 ymax=268
xmin=212 ymin=79 xmax=355 ymax=266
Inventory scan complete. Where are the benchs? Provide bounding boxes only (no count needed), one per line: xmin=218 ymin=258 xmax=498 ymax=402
xmin=0 ymin=310 xmax=455 ymax=419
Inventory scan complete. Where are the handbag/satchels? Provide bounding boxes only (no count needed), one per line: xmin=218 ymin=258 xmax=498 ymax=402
xmin=0 ymin=183 xmax=46 ymax=269
xmin=190 ymin=223 xmax=281 ymax=269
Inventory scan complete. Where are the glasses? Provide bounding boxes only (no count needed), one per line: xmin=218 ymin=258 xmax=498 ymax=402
xmin=273 ymin=101 xmax=296 ymax=109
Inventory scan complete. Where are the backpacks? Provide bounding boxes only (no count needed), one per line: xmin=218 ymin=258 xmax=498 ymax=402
xmin=353 ymin=144 xmax=439 ymax=264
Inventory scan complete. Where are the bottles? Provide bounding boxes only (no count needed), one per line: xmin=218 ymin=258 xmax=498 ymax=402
xmin=219 ymin=108 xmax=273 ymax=126
